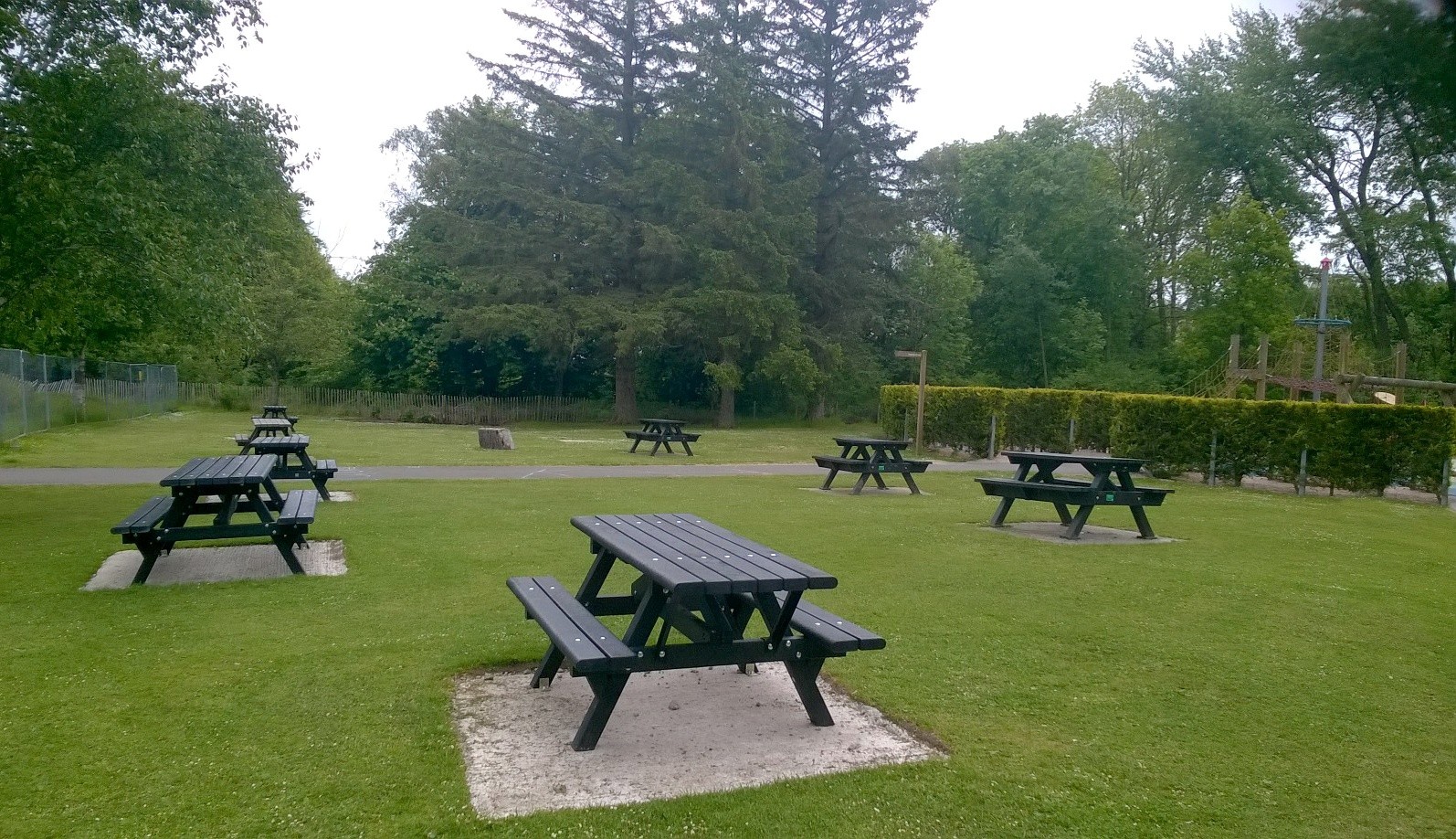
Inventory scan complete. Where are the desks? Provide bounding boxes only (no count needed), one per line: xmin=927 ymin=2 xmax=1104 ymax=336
xmin=249 ymin=434 xmax=331 ymax=502
xmin=986 ymin=448 xmax=1158 ymax=540
xmin=262 ymin=403 xmax=295 ymax=432
xmin=818 ymin=436 xmax=921 ymax=496
xmin=128 ymin=453 xmax=308 ymax=585
xmin=628 ymin=418 xmax=694 ymax=457
xmin=239 ymin=416 xmax=293 ymax=455
xmin=526 ymin=509 xmax=835 ymax=749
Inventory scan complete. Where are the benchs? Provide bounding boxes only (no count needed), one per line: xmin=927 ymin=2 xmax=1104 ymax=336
xmin=251 ymin=414 xmax=260 ymax=418
xmin=666 ymin=433 xmax=702 ymax=443
xmin=314 ymin=454 xmax=339 ymax=487
xmin=276 ymin=487 xmax=321 ymax=548
xmin=811 ymin=454 xmax=870 ymax=476
xmin=622 ymin=429 xmax=660 ymax=443
xmin=235 ymin=433 xmax=249 ymax=443
xmin=974 ymin=474 xmax=1096 ymax=508
xmin=109 ymin=494 xmax=175 ymax=562
xmin=507 ymin=576 xmax=639 ymax=674
xmin=289 ymin=416 xmax=300 ymax=425
xmin=1053 ymin=477 xmax=1175 ymax=506
xmin=743 ymin=592 xmax=886 ymax=654
xmin=879 ymin=456 xmax=934 ymax=473
